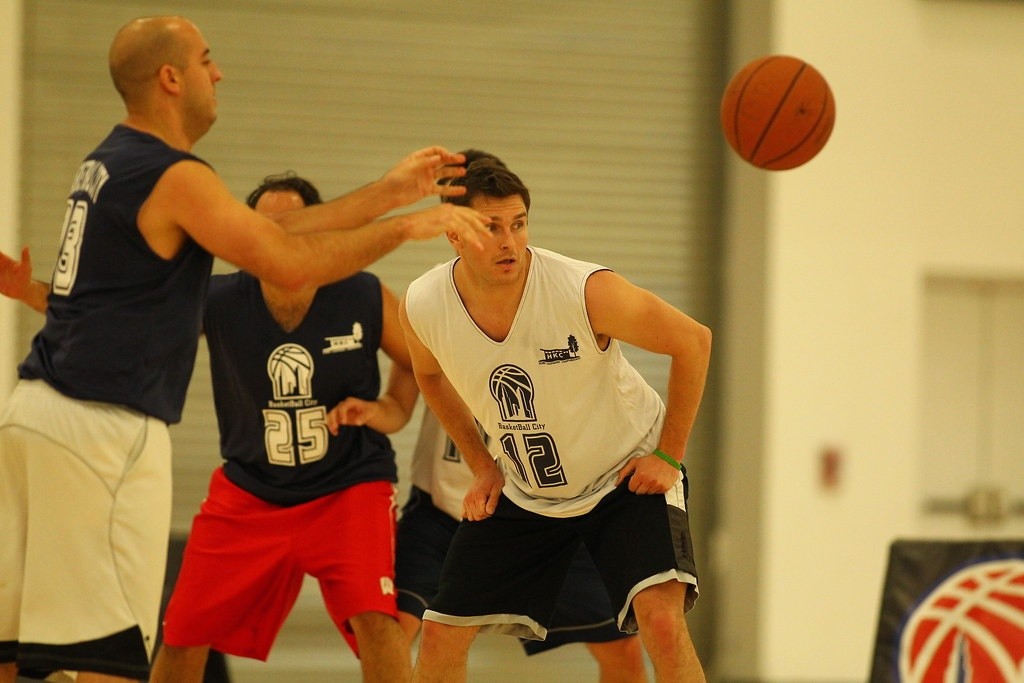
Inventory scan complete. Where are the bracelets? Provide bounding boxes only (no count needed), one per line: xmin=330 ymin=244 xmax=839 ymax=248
xmin=654 ymin=449 xmax=682 ymax=471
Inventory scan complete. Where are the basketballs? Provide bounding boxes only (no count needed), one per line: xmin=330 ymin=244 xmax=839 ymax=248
xmin=720 ymin=55 xmax=836 ymax=172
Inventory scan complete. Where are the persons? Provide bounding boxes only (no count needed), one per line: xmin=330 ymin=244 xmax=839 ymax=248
xmin=0 ymin=15 xmax=492 ymax=683
xmin=392 ymin=148 xmax=648 ymax=682
xmin=396 ymin=159 xmax=713 ymax=682
xmin=1 ymin=171 xmax=420 ymax=683
